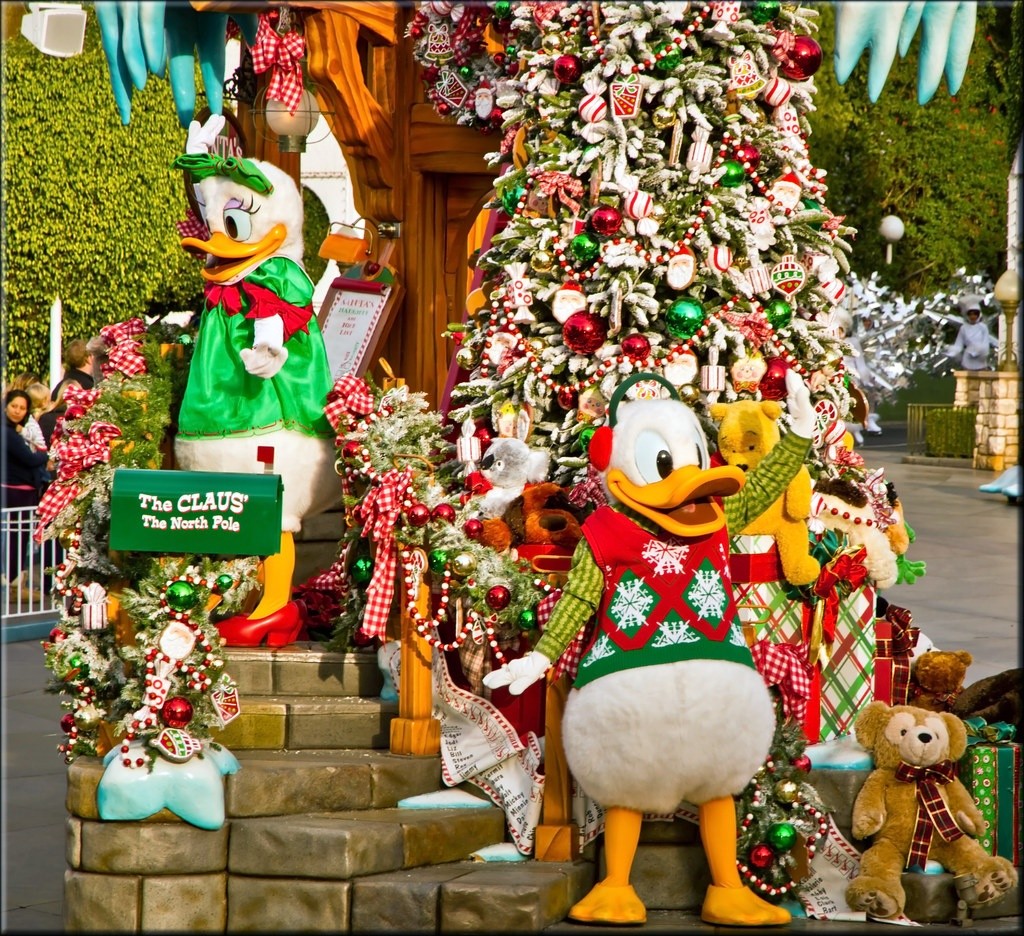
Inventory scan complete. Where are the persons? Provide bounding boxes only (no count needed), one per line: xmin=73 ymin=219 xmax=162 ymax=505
xmin=0 ymin=336 xmax=107 ymax=604
xmin=838 ymin=294 xmax=989 ymax=444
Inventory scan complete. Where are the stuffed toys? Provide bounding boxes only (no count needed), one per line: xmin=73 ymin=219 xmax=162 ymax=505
xmin=845 ymin=699 xmax=1020 ymax=921
xmin=466 ymin=437 xmax=548 ymax=520
xmin=911 ymin=650 xmax=971 ymax=706
xmin=480 ymin=480 xmax=584 ymax=547
xmin=482 ymin=366 xmax=819 ymax=927
xmin=174 ymin=114 xmax=342 ymax=649
xmin=710 ymin=397 xmax=821 ymax=585
xmin=817 ymin=468 xmax=940 ymax=659
